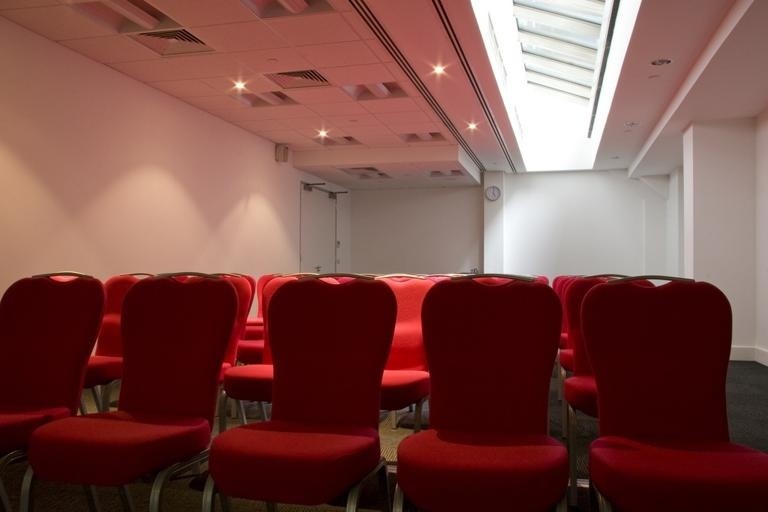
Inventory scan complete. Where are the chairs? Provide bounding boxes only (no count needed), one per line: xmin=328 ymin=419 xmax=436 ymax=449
xmin=0 ymin=265 xmax=768 ymax=512
